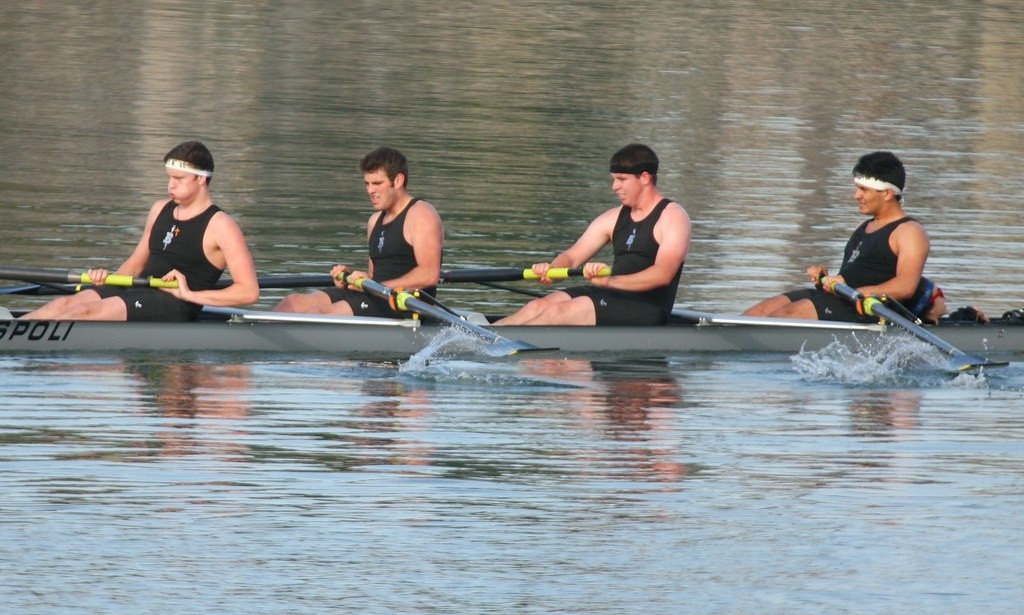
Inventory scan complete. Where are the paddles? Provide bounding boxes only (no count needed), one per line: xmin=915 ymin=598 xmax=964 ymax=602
xmin=812 ymin=268 xmax=1010 ymax=371
xmin=2 ymin=272 xmax=351 ymax=295
xmin=0 ymin=265 xmax=180 ymax=292
xmin=336 ymin=269 xmax=560 ymax=354
xmin=212 ymin=266 xmax=619 ymax=289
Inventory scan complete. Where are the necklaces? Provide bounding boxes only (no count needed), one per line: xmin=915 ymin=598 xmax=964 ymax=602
xmin=174 ymin=203 xmax=211 ymax=236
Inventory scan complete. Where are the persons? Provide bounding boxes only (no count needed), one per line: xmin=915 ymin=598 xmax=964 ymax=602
xmin=902 ymin=277 xmax=990 ymax=325
xmin=737 ymin=151 xmax=930 ymax=323
xmin=269 ymin=147 xmax=444 ymax=321
xmin=17 ymin=140 xmax=259 ymax=321
xmin=489 ymin=143 xmax=691 ymax=326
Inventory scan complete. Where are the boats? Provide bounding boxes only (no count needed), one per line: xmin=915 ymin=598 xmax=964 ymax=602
xmin=0 ymin=265 xmax=1023 ymax=370
